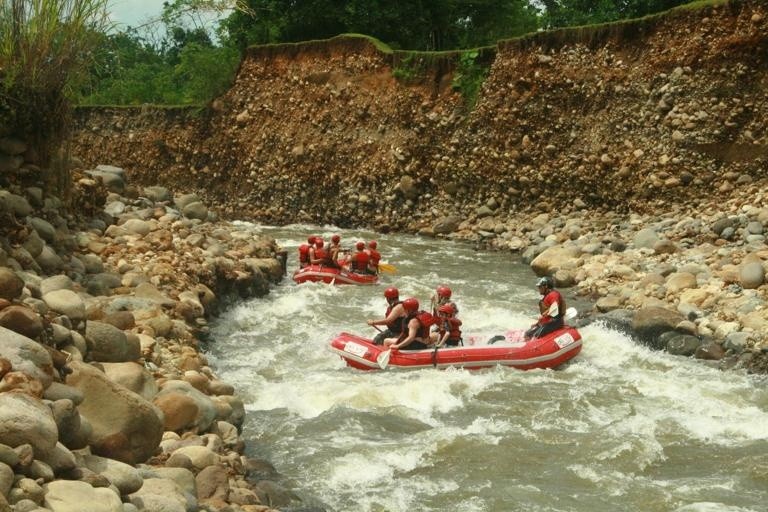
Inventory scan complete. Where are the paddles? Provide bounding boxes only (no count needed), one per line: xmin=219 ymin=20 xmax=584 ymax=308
xmin=564 ymin=307 xmax=578 ymax=323
xmin=377 ymin=263 xmax=396 ymax=272
xmin=330 ymin=253 xmax=349 ymax=288
xmin=535 ymin=301 xmax=560 ymax=325
xmin=377 ymin=330 xmax=403 ymax=372
xmin=431 ymin=334 xmax=440 ymax=368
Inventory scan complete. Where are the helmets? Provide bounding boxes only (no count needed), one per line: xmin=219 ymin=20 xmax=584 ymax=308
xmin=385 ymin=287 xmax=399 ymax=298
xmin=369 ymin=240 xmax=377 ymax=250
xmin=357 ymin=242 xmax=365 ymax=249
xmin=436 ymin=287 xmax=453 ymax=298
xmin=403 ymin=298 xmax=420 ymax=311
xmin=439 ymin=305 xmax=454 ymax=315
xmin=332 ymin=235 xmax=340 ymax=242
xmin=308 ymin=235 xmax=316 ymax=244
xmin=315 ymin=238 xmax=324 ymax=247
xmin=537 ymin=276 xmax=554 ymax=288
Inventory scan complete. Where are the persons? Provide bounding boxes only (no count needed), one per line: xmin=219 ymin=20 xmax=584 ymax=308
xmin=429 ymin=287 xmax=457 ymax=331
xmin=366 ymin=287 xmax=405 ymax=345
xmin=379 ymin=297 xmax=430 ymax=350
xmin=433 ymin=304 xmax=461 ymax=350
xmin=298 ymin=235 xmax=381 ymax=277
xmin=522 ymin=275 xmax=566 ymax=343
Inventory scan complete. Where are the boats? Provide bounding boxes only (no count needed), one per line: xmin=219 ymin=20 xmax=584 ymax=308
xmin=326 ymin=320 xmax=583 ymax=370
xmin=292 ymin=264 xmax=378 ymax=287
